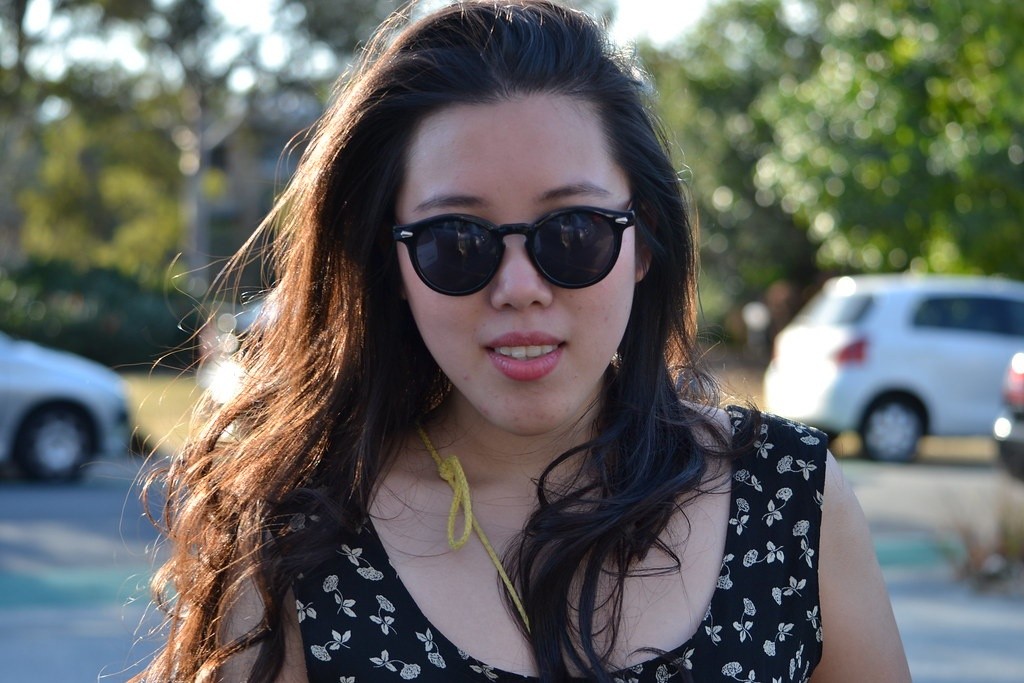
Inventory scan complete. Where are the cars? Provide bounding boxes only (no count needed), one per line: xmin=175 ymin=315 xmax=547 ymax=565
xmin=1 ymin=330 xmax=138 ymax=484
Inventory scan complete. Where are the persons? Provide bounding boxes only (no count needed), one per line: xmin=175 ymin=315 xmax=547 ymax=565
xmin=121 ymin=0 xmax=914 ymax=683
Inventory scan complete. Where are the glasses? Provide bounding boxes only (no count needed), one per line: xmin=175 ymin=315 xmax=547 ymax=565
xmin=392 ymin=201 xmax=636 ymax=297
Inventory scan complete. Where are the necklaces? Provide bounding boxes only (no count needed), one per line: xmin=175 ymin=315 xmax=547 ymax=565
xmin=412 ymin=418 xmax=530 ymax=636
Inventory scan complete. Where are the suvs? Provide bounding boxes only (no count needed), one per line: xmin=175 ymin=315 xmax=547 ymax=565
xmin=760 ymin=274 xmax=1022 ymax=464
